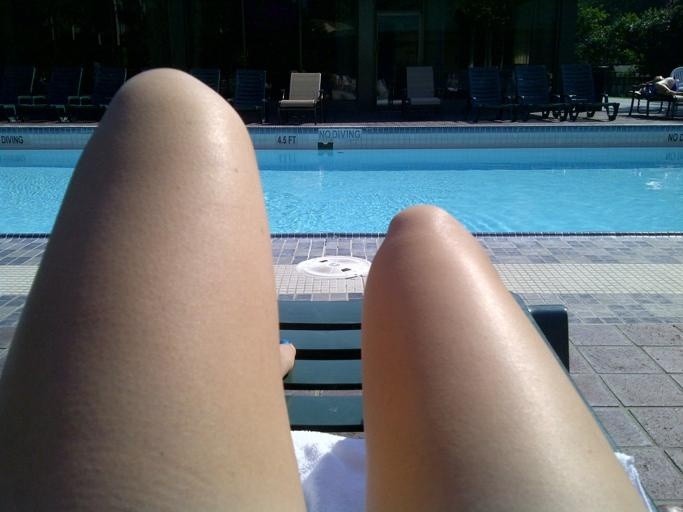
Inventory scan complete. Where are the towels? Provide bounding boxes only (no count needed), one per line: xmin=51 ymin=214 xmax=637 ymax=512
xmin=290 ymin=431 xmax=654 ymax=512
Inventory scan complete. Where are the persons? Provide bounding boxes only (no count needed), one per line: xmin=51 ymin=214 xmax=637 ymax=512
xmin=650 ymin=74 xmax=682 ymax=99
xmin=0 ymin=64 xmax=656 ymax=510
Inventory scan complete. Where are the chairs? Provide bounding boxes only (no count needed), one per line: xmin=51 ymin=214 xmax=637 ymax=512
xmin=560 ymin=64 xmax=621 ymax=123
xmin=506 ymin=64 xmax=570 ymax=123
xmin=625 ymin=66 xmax=683 ymax=119
xmin=402 ymin=64 xmax=445 ymax=120
xmin=227 ymin=67 xmax=268 ymax=124
xmin=257 ymin=290 xmax=659 ymax=512
xmin=2 ymin=64 xmax=128 ymax=122
xmin=186 ymin=66 xmax=221 ymax=91
xmin=466 ymin=64 xmax=517 ymax=122
xmin=277 ymin=71 xmax=322 ymax=123
